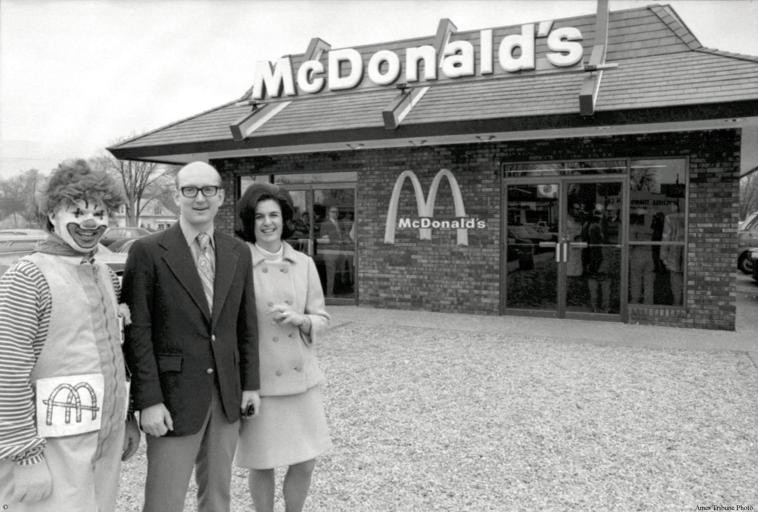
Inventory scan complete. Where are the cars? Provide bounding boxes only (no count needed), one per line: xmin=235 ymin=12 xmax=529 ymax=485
xmin=507 ymin=221 xmax=556 ymax=258
xmin=735 ymin=210 xmax=757 ymax=284
xmin=0 ymin=224 xmax=155 ymax=290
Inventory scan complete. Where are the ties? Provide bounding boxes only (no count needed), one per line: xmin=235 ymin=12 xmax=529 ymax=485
xmin=196 ymin=233 xmax=216 ymax=318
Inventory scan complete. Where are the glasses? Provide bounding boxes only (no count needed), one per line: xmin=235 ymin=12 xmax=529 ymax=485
xmin=180 ymin=186 xmax=223 ymax=197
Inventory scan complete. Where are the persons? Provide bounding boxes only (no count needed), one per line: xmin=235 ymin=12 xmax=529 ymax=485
xmin=121 ymin=160 xmax=259 ymax=512
xmin=290 ymin=203 xmax=352 ymax=297
xmin=0 ymin=159 xmax=140 ymax=512
xmin=236 ymin=182 xmax=331 ymax=512
xmin=511 ymin=189 xmax=687 ymax=317
